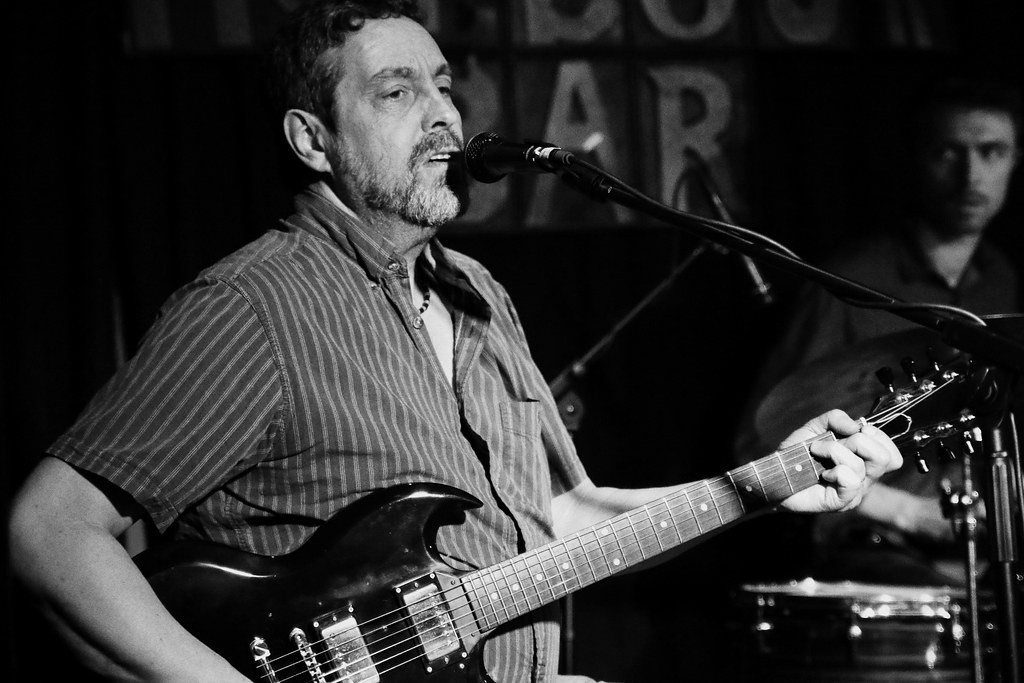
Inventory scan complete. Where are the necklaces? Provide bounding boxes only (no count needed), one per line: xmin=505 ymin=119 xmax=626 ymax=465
xmin=415 ymin=283 xmax=431 ymax=315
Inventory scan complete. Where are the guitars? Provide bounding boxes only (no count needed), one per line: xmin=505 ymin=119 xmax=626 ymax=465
xmin=129 ymin=343 xmax=997 ymax=683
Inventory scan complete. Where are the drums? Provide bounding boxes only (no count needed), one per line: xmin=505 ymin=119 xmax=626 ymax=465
xmin=742 ymin=578 xmax=1000 ymax=683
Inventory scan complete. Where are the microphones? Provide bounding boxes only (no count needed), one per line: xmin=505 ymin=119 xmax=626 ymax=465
xmin=464 ymin=130 xmax=575 ymax=184
xmin=712 ymin=193 xmax=774 ymax=306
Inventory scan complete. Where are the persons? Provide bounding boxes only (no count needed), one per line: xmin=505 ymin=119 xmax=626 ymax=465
xmin=725 ymin=90 xmax=1023 ymax=584
xmin=7 ymin=0 xmax=903 ymax=683
